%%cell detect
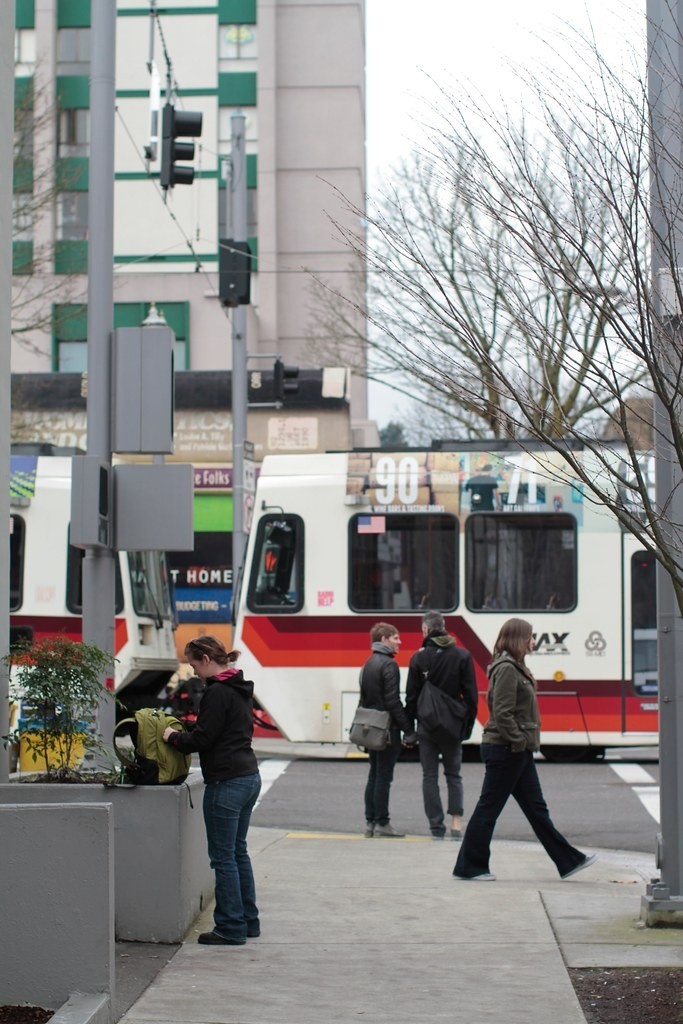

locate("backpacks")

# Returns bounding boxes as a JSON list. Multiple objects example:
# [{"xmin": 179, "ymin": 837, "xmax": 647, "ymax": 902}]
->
[{"xmin": 113, "ymin": 708, "xmax": 191, "ymax": 785}]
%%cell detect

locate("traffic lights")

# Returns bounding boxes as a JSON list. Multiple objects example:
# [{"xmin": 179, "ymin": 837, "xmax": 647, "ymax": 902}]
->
[{"xmin": 158, "ymin": 104, "xmax": 203, "ymax": 191}]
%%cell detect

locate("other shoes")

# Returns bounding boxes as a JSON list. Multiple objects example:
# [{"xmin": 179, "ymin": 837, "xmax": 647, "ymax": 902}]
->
[
  {"xmin": 198, "ymin": 932, "xmax": 247, "ymax": 945},
  {"xmin": 365, "ymin": 822, "xmax": 373, "ymax": 838},
  {"xmin": 561, "ymin": 854, "xmax": 598, "ymax": 879},
  {"xmin": 247, "ymin": 929, "xmax": 260, "ymax": 937},
  {"xmin": 450, "ymin": 829, "xmax": 462, "ymax": 841},
  {"xmin": 452, "ymin": 873, "xmax": 496, "ymax": 881},
  {"xmin": 374, "ymin": 822, "xmax": 406, "ymax": 838},
  {"xmin": 432, "ymin": 834, "xmax": 444, "ymax": 840}
]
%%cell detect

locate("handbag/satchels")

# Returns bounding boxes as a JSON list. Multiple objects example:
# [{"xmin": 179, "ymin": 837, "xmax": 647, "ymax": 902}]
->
[
  {"xmin": 349, "ymin": 706, "xmax": 392, "ymax": 752},
  {"xmin": 416, "ymin": 680, "xmax": 467, "ymax": 746}
]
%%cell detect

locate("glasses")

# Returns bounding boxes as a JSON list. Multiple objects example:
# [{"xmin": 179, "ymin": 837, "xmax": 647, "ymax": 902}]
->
[{"xmin": 189, "ymin": 641, "xmax": 211, "ymax": 660}]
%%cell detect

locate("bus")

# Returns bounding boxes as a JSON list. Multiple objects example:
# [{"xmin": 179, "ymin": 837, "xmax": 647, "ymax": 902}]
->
[
  {"xmin": 229, "ymin": 449, "xmax": 660, "ymax": 766},
  {"xmin": 10, "ymin": 455, "xmax": 180, "ymax": 737}
]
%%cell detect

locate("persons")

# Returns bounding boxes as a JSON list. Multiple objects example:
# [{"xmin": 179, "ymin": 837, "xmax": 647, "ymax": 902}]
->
[
  {"xmin": 465, "ymin": 464, "xmax": 500, "ymax": 513},
  {"xmin": 453, "ymin": 618, "xmax": 598, "ymax": 881},
  {"xmin": 163, "ymin": 636, "xmax": 261, "ymax": 944},
  {"xmin": 404, "ymin": 610, "xmax": 478, "ymax": 841},
  {"xmin": 358, "ymin": 623, "xmax": 410, "ymax": 838}
]
